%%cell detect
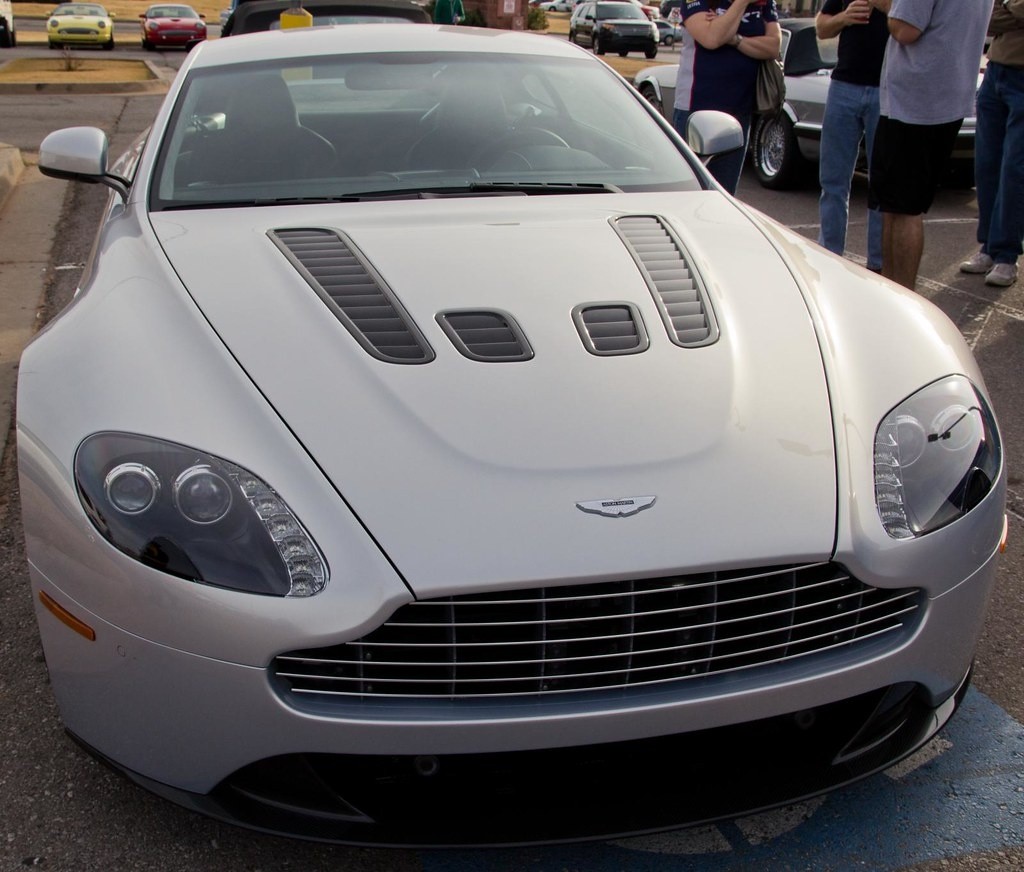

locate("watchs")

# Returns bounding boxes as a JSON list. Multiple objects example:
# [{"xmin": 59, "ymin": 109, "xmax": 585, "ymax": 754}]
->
[{"xmin": 733, "ymin": 34, "xmax": 741, "ymax": 48}]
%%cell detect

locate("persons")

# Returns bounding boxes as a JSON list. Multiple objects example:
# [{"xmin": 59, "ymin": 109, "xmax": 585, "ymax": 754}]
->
[
  {"xmin": 669, "ymin": 0, "xmax": 783, "ymax": 201},
  {"xmin": 814, "ymin": 0, "xmax": 1024, "ymax": 292}
]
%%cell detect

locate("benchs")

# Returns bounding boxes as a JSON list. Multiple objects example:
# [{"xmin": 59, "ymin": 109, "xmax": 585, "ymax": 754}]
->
[{"xmin": 295, "ymin": 110, "xmax": 430, "ymax": 177}]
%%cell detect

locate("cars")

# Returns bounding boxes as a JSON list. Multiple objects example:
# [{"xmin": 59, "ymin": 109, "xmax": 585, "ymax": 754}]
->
[
  {"xmin": 45, "ymin": 4, "xmax": 116, "ymax": 51},
  {"xmin": 220, "ymin": 0, "xmax": 434, "ymax": 39},
  {"xmin": 632, "ymin": 18, "xmax": 892, "ymax": 190},
  {"xmin": 139, "ymin": 4, "xmax": 207, "ymax": 52},
  {"xmin": 570, "ymin": 1, "xmax": 660, "ymax": 59},
  {"xmin": 15, "ymin": 26, "xmax": 1006, "ymax": 850},
  {"xmin": 654, "ymin": 21, "xmax": 685, "ymax": 46},
  {"xmin": 538, "ymin": 0, "xmax": 573, "ymax": 12}
]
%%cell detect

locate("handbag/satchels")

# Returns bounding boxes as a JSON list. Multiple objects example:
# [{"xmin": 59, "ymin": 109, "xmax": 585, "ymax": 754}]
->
[{"xmin": 756, "ymin": 58, "xmax": 787, "ymax": 115}]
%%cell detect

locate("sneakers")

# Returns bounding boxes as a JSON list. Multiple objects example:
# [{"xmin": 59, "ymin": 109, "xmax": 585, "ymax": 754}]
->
[
  {"xmin": 959, "ymin": 251, "xmax": 994, "ymax": 273},
  {"xmin": 985, "ymin": 262, "xmax": 1018, "ymax": 286}
]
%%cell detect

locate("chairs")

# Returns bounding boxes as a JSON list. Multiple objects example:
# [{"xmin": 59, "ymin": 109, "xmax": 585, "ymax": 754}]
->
[
  {"xmin": 186, "ymin": 74, "xmax": 338, "ymax": 184},
  {"xmin": 403, "ymin": 62, "xmax": 545, "ymax": 170}
]
